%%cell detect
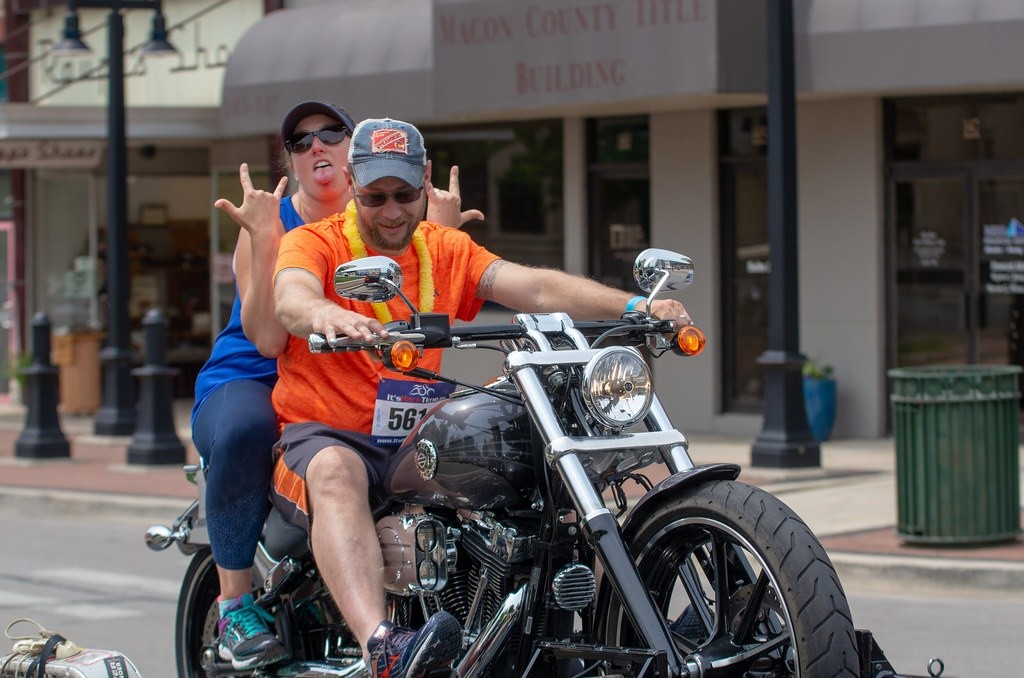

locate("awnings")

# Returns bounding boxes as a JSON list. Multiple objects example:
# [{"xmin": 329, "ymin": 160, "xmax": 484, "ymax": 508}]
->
[{"xmin": 220, "ymin": 0, "xmax": 1023, "ymax": 135}]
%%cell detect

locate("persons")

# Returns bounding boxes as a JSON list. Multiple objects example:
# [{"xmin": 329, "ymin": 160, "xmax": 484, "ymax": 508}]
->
[
  {"xmin": 190, "ymin": 100, "xmax": 485, "ymax": 669},
  {"xmin": 271, "ymin": 115, "xmax": 696, "ymax": 678}
]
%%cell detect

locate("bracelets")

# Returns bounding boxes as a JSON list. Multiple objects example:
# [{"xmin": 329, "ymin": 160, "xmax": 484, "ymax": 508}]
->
[{"xmin": 626, "ymin": 296, "xmax": 647, "ymax": 311}]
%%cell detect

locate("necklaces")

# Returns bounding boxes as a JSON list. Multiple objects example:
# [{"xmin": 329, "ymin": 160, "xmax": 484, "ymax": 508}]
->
[{"xmin": 341, "ymin": 197, "xmax": 434, "ymax": 326}]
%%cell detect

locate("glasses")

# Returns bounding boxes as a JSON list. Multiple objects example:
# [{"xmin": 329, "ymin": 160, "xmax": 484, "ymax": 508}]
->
[
  {"xmin": 286, "ymin": 123, "xmax": 353, "ymax": 154},
  {"xmin": 351, "ymin": 172, "xmax": 427, "ymax": 207}
]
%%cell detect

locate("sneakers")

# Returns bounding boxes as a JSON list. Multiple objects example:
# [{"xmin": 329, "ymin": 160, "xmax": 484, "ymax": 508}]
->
[
  {"xmin": 366, "ymin": 611, "xmax": 461, "ymax": 678},
  {"xmin": 214, "ymin": 592, "xmax": 291, "ymax": 669}
]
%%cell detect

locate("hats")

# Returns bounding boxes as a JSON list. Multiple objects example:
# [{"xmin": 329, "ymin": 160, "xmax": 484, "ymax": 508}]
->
[
  {"xmin": 347, "ymin": 117, "xmax": 427, "ymax": 190},
  {"xmin": 280, "ymin": 101, "xmax": 358, "ymax": 154}
]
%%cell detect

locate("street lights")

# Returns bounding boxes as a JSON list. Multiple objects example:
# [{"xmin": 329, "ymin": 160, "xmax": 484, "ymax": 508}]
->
[{"xmin": 52, "ymin": 0, "xmax": 178, "ymax": 435}]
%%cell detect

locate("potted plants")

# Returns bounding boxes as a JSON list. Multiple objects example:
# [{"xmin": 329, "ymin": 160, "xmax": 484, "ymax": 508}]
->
[{"xmin": 801, "ymin": 364, "xmax": 837, "ymax": 441}]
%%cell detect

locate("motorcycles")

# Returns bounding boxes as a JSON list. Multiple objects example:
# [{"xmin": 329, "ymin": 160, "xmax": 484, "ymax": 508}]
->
[{"xmin": 0, "ymin": 248, "xmax": 944, "ymax": 678}]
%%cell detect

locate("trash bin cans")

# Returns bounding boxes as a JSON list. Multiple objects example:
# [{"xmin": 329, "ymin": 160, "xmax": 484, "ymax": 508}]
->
[{"xmin": 887, "ymin": 365, "xmax": 1024, "ymax": 547}]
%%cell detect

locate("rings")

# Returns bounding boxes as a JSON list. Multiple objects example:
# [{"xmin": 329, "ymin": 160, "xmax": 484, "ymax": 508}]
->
[{"xmin": 680, "ymin": 315, "xmax": 686, "ymax": 318}]
